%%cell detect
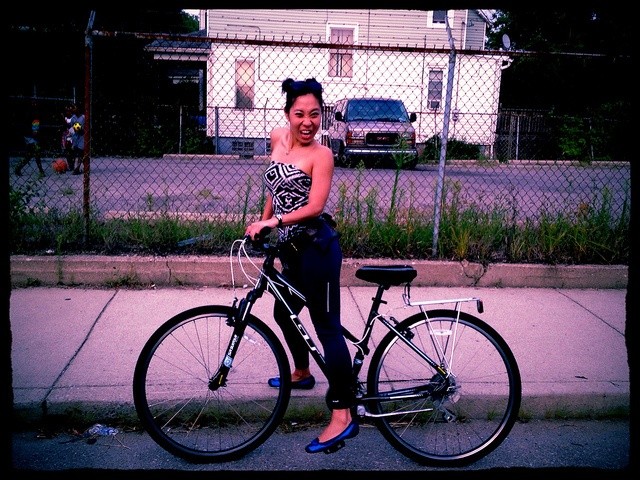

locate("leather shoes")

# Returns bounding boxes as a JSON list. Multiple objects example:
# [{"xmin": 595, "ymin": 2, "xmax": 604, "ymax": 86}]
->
[
  {"xmin": 305, "ymin": 417, "xmax": 359, "ymax": 453},
  {"xmin": 269, "ymin": 375, "xmax": 315, "ymax": 389}
]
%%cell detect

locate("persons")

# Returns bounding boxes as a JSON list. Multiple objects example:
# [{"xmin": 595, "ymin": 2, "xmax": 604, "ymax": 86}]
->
[
  {"xmin": 60, "ymin": 111, "xmax": 75, "ymax": 172},
  {"xmin": 70, "ymin": 102, "xmax": 93, "ymax": 175},
  {"xmin": 242, "ymin": 76, "xmax": 361, "ymax": 456},
  {"xmin": 14, "ymin": 100, "xmax": 47, "ymax": 177}
]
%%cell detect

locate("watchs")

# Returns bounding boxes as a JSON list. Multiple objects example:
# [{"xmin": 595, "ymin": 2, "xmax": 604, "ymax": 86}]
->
[{"xmin": 274, "ymin": 214, "xmax": 283, "ymax": 226}]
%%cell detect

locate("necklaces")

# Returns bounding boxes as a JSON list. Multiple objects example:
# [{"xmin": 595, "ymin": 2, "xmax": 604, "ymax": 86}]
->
[{"xmin": 286, "ymin": 140, "xmax": 299, "ymax": 156}]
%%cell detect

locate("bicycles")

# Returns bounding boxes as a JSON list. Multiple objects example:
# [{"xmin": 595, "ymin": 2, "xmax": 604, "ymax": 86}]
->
[{"xmin": 133, "ymin": 226, "xmax": 521, "ymax": 467}]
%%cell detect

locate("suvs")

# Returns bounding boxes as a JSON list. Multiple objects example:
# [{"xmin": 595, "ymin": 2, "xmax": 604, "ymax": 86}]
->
[{"xmin": 328, "ymin": 98, "xmax": 418, "ymax": 170}]
